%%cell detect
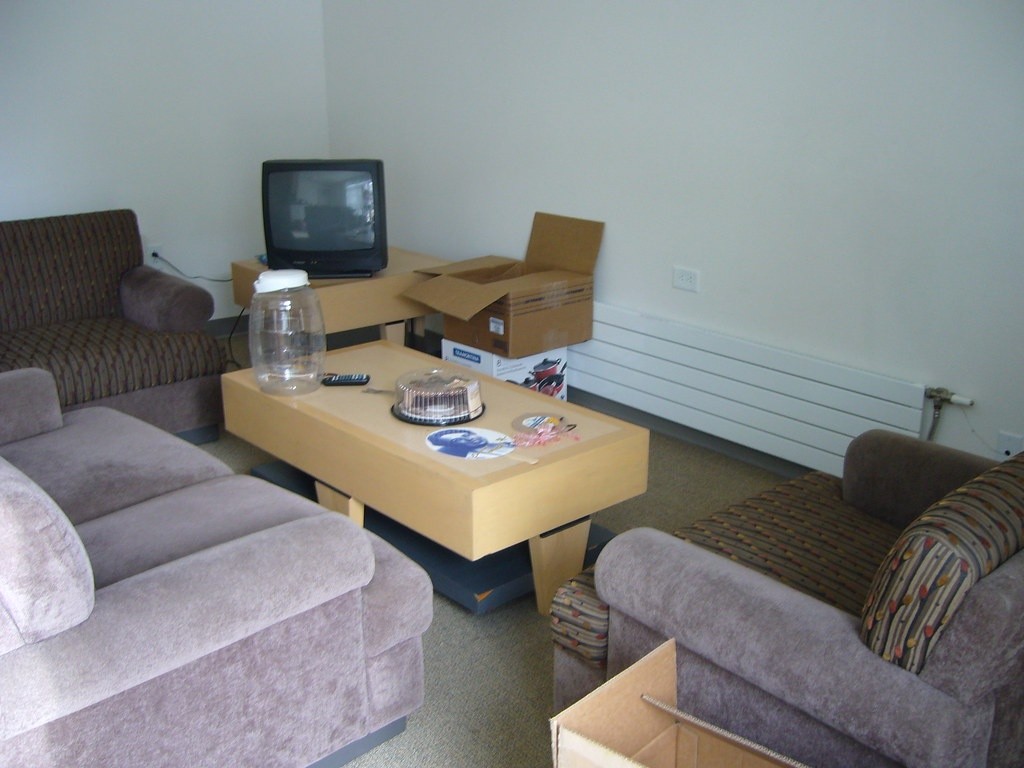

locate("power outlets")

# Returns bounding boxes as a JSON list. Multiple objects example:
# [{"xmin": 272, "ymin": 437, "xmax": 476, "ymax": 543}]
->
[
  {"xmin": 673, "ymin": 266, "xmax": 699, "ymax": 291},
  {"xmin": 146, "ymin": 246, "xmax": 163, "ymax": 272}
]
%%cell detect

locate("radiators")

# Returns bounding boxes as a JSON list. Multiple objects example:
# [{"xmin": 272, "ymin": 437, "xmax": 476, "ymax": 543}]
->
[{"xmin": 568, "ymin": 302, "xmax": 973, "ymax": 477}]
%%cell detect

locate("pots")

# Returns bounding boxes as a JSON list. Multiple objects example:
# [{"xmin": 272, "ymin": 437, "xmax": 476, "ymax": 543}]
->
[{"xmin": 505, "ymin": 358, "xmax": 567, "ymax": 397}]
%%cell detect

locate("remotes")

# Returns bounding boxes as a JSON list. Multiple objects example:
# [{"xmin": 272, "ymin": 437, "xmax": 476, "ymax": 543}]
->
[{"xmin": 322, "ymin": 374, "xmax": 371, "ymax": 386}]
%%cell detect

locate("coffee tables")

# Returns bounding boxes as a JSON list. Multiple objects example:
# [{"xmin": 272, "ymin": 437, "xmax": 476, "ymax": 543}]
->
[{"xmin": 221, "ymin": 340, "xmax": 649, "ymax": 614}]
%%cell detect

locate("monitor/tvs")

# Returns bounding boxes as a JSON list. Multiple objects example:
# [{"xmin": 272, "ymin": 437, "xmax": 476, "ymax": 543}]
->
[{"xmin": 262, "ymin": 157, "xmax": 390, "ymax": 278}]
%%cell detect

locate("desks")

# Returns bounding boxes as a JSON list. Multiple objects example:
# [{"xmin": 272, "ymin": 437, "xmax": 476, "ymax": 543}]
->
[{"xmin": 232, "ymin": 246, "xmax": 451, "ymax": 347}]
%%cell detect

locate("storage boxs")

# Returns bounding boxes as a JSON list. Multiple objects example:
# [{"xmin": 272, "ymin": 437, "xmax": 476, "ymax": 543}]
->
[
  {"xmin": 442, "ymin": 338, "xmax": 568, "ymax": 402},
  {"xmin": 401, "ymin": 212, "xmax": 604, "ymax": 359},
  {"xmin": 549, "ymin": 637, "xmax": 810, "ymax": 768}
]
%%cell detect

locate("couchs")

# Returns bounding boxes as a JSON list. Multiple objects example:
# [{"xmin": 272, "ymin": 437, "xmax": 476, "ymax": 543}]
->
[
  {"xmin": 548, "ymin": 428, "xmax": 1024, "ymax": 768},
  {"xmin": 0, "ymin": 368, "xmax": 433, "ymax": 767},
  {"xmin": 0, "ymin": 209, "xmax": 225, "ymax": 444}
]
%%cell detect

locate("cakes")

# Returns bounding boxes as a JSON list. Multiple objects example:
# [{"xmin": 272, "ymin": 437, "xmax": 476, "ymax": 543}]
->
[{"xmin": 399, "ymin": 375, "xmax": 483, "ymax": 422}]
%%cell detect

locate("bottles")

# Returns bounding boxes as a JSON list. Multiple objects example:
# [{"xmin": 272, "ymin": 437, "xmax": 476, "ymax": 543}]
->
[{"xmin": 247, "ymin": 269, "xmax": 328, "ymax": 399}]
